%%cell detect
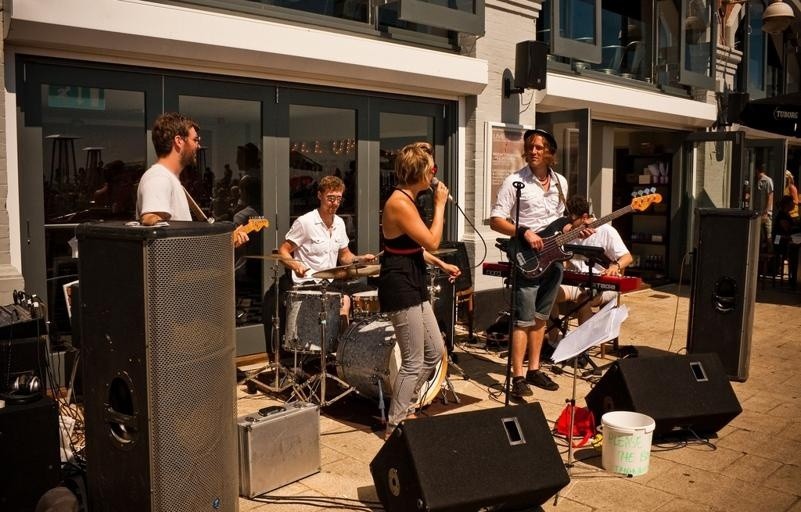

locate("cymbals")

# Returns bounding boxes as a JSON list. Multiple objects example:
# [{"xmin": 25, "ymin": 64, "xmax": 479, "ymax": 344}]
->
[
  {"xmin": 313, "ymin": 265, "xmax": 380, "ymax": 278},
  {"xmin": 427, "ymin": 249, "xmax": 457, "ymax": 256},
  {"xmin": 353, "ymin": 289, "xmax": 379, "ymax": 314}
]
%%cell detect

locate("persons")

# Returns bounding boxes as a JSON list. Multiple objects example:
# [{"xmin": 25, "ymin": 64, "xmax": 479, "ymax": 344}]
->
[
  {"xmin": 377, "ymin": 141, "xmax": 464, "ymax": 442},
  {"xmin": 784, "ymin": 168, "xmax": 800, "ymax": 223},
  {"xmin": 772, "ymin": 196, "xmax": 801, "ymax": 288},
  {"xmin": 183, "ymin": 163, "xmax": 241, "ymax": 221},
  {"xmin": 135, "ymin": 110, "xmax": 251, "ymax": 249},
  {"xmin": 491, "ymin": 126, "xmax": 598, "ymax": 400},
  {"xmin": 278, "ymin": 174, "xmax": 380, "ymax": 335},
  {"xmin": 289, "ymin": 160, "xmax": 357, "ymax": 216},
  {"xmin": 233, "ymin": 143, "xmax": 262, "ymax": 223},
  {"xmin": 93, "ymin": 161, "xmax": 138, "ymax": 220},
  {"xmin": 753, "ymin": 161, "xmax": 777, "ymax": 255},
  {"xmin": 546, "ymin": 193, "xmax": 636, "ymax": 369}
]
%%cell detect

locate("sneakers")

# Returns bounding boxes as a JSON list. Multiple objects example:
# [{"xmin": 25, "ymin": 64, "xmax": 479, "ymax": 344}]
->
[{"xmin": 512, "ymin": 370, "xmax": 559, "ymax": 395}]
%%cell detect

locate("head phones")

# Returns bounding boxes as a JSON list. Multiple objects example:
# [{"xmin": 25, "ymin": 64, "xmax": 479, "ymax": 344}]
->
[{"xmin": 0, "ymin": 375, "xmax": 42, "ymax": 404}]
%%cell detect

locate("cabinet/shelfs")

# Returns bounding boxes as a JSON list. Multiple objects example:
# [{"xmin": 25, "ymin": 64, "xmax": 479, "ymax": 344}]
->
[{"xmin": 621, "ymin": 149, "xmax": 680, "ymax": 283}]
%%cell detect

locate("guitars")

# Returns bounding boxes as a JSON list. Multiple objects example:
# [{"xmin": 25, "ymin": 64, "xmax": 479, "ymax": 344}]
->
[
  {"xmin": 234, "ymin": 216, "xmax": 269, "ymax": 245},
  {"xmin": 506, "ymin": 187, "xmax": 662, "ymax": 279}
]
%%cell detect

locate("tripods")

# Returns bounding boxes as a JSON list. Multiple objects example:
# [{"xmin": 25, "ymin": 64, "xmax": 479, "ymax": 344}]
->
[
  {"xmin": 240, "ymin": 260, "xmax": 469, "ymax": 424},
  {"xmin": 553, "ymin": 357, "xmax": 633, "ymax": 507}
]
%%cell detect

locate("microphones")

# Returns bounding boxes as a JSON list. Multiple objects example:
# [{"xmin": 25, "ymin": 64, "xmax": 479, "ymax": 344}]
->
[{"xmin": 432, "ymin": 177, "xmax": 457, "ymax": 206}]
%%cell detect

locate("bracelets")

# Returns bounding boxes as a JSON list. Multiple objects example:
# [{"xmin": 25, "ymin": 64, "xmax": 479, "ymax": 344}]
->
[
  {"xmin": 614, "ymin": 261, "xmax": 621, "ymax": 271},
  {"xmin": 519, "ymin": 225, "xmax": 529, "ymax": 236}
]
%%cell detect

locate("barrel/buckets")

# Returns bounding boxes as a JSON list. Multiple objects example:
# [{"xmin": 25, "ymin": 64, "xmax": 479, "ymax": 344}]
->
[{"xmin": 600, "ymin": 410, "xmax": 655, "ymax": 476}]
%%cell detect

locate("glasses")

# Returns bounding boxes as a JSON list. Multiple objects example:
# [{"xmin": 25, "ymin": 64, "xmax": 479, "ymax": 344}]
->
[
  {"xmin": 430, "ymin": 165, "xmax": 438, "ymax": 174},
  {"xmin": 327, "ymin": 195, "xmax": 344, "ymax": 202}
]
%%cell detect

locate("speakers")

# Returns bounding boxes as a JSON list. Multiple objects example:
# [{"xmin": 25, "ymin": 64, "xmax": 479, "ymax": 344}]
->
[
  {"xmin": 515, "ymin": 40, "xmax": 547, "ymax": 90},
  {"xmin": 584, "ymin": 350, "xmax": 743, "ymax": 445},
  {"xmin": 369, "ymin": 402, "xmax": 570, "ymax": 512},
  {"xmin": 0, "ymin": 396, "xmax": 62, "ymax": 512},
  {"xmin": 686, "ymin": 206, "xmax": 762, "ymax": 382},
  {"xmin": 727, "ymin": 91, "xmax": 749, "ymax": 120},
  {"xmin": 436, "ymin": 240, "xmax": 472, "ymax": 292},
  {"xmin": 0, "ymin": 334, "xmax": 47, "ymax": 400},
  {"xmin": 77, "ymin": 220, "xmax": 238, "ymax": 512}
]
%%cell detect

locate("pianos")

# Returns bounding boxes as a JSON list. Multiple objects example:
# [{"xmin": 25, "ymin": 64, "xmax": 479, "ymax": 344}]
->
[{"xmin": 484, "ymin": 261, "xmax": 640, "ymax": 292}]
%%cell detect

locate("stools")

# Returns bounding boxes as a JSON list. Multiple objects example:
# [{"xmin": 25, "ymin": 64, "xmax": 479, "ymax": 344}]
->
[
  {"xmin": 557, "ymin": 290, "xmax": 621, "ymax": 360},
  {"xmin": 455, "ymin": 286, "xmax": 478, "ymax": 339}
]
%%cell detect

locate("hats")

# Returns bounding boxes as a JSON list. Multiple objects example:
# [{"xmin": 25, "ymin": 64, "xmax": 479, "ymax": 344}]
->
[{"xmin": 524, "ymin": 127, "xmax": 557, "ymax": 148}]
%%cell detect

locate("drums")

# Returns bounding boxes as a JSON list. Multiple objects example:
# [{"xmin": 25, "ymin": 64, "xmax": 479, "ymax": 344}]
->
[
  {"xmin": 283, "ymin": 290, "xmax": 343, "ymax": 356},
  {"xmin": 336, "ymin": 314, "xmax": 449, "ymax": 407}
]
